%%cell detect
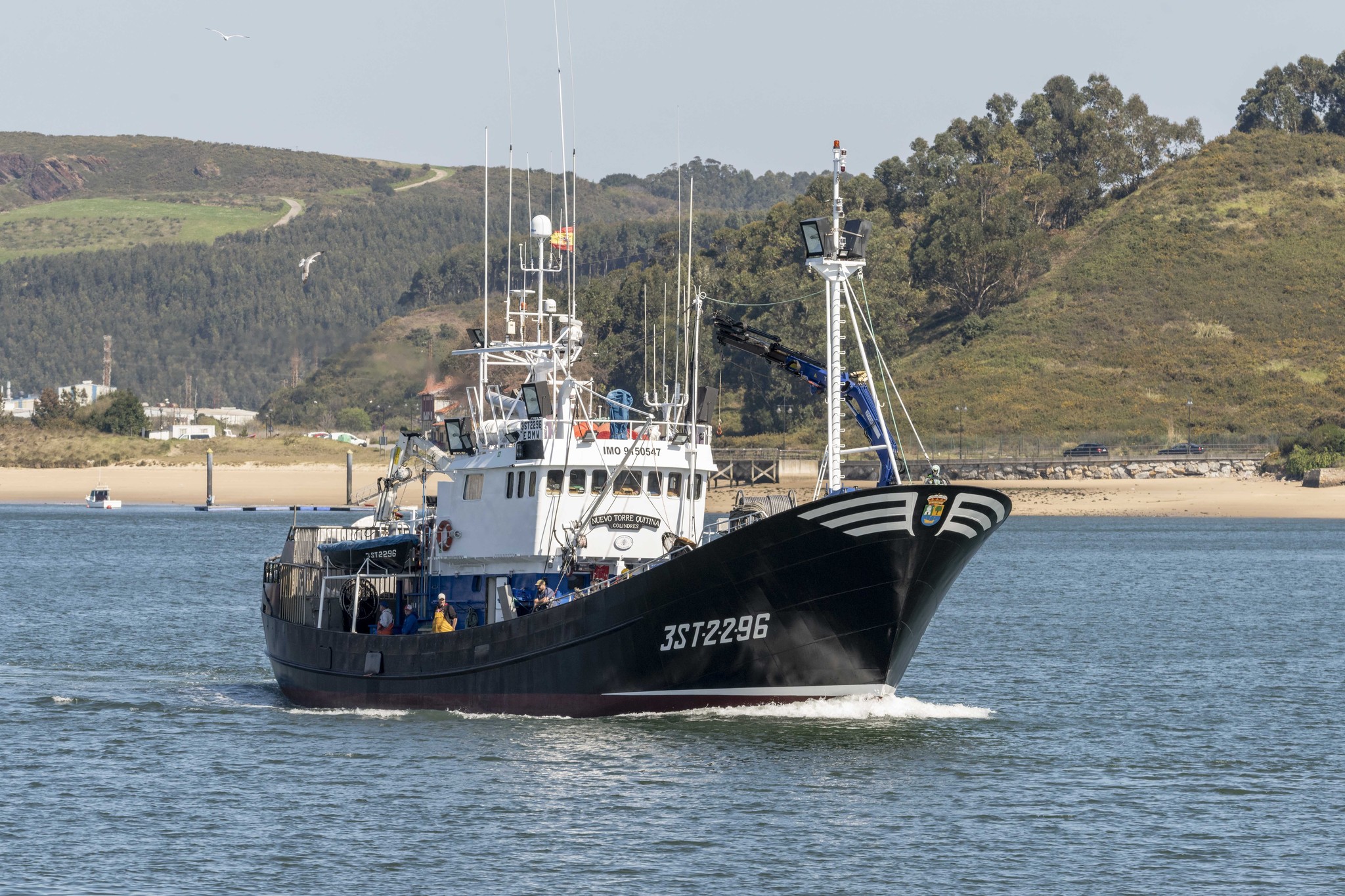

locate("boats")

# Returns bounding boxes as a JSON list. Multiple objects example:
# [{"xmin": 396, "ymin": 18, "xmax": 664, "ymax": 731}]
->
[
  {"xmin": 86, "ymin": 459, "xmax": 122, "ymax": 509},
  {"xmin": 259, "ymin": 4, "xmax": 1015, "ymax": 704}
]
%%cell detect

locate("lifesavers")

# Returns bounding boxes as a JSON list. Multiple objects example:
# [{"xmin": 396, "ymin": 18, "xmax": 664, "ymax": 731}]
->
[
  {"xmin": 437, "ymin": 520, "xmax": 452, "ymax": 551},
  {"xmin": 416, "ymin": 523, "xmax": 431, "ymax": 553}
]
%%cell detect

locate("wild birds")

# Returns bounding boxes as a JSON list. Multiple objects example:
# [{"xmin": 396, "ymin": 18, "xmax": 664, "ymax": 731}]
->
[
  {"xmin": 298, "ymin": 251, "xmax": 325, "ymax": 285},
  {"xmin": 204, "ymin": 28, "xmax": 250, "ymax": 41}
]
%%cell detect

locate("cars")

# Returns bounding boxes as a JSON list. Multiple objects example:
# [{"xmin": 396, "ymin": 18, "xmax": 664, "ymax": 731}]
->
[
  {"xmin": 1158, "ymin": 442, "xmax": 1205, "ymax": 455},
  {"xmin": 303, "ymin": 431, "xmax": 366, "ymax": 447}
]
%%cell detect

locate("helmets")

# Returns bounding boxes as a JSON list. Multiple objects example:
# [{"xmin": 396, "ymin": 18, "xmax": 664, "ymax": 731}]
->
[{"xmin": 621, "ymin": 569, "xmax": 632, "ymax": 577}]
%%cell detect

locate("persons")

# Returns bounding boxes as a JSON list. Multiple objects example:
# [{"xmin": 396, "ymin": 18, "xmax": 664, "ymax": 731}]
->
[
  {"xmin": 401, "ymin": 604, "xmax": 418, "ymax": 635},
  {"xmin": 923, "ymin": 465, "xmax": 946, "ymax": 484},
  {"xmin": 377, "ymin": 601, "xmax": 394, "ymax": 635},
  {"xmin": 534, "ymin": 579, "xmax": 557, "ymax": 612},
  {"xmin": 432, "ymin": 593, "xmax": 457, "ymax": 634}
]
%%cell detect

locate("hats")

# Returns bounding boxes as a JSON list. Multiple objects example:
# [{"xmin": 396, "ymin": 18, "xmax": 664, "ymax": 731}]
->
[
  {"xmin": 931, "ymin": 466, "xmax": 939, "ymax": 470},
  {"xmin": 379, "ymin": 601, "xmax": 388, "ymax": 608},
  {"xmin": 534, "ymin": 580, "xmax": 545, "ymax": 586},
  {"xmin": 405, "ymin": 604, "xmax": 412, "ymax": 610},
  {"xmin": 438, "ymin": 593, "xmax": 445, "ymax": 600}
]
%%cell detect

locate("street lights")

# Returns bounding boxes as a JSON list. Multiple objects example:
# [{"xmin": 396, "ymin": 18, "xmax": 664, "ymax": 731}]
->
[
  {"xmin": 775, "ymin": 396, "xmax": 794, "ymax": 446},
  {"xmin": 954, "ymin": 396, "xmax": 968, "ymax": 458},
  {"xmin": 1186, "ymin": 389, "xmax": 1193, "ymax": 455}
]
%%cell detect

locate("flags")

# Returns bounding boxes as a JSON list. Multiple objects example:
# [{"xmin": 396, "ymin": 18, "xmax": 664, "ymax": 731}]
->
[{"xmin": 548, "ymin": 226, "xmax": 575, "ymax": 252}]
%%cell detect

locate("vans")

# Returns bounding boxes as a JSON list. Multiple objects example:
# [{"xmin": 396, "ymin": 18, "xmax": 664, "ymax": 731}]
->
[{"xmin": 178, "ymin": 433, "xmax": 211, "ymax": 443}]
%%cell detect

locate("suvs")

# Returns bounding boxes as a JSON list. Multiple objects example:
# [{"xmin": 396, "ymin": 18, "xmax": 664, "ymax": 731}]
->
[{"xmin": 1063, "ymin": 443, "xmax": 1108, "ymax": 458}]
